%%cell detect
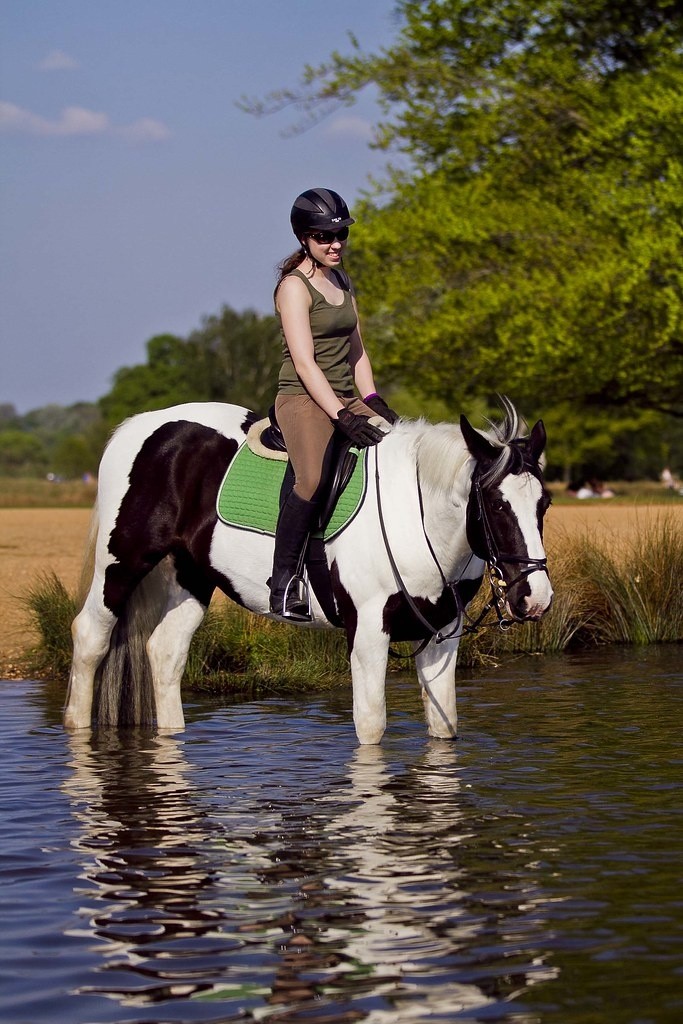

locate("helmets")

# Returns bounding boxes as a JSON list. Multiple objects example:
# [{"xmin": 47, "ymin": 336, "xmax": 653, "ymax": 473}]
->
[{"xmin": 290, "ymin": 188, "xmax": 355, "ymax": 238}]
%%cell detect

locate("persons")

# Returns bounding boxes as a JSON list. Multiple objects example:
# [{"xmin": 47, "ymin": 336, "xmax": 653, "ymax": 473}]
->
[{"xmin": 267, "ymin": 187, "xmax": 402, "ymax": 622}]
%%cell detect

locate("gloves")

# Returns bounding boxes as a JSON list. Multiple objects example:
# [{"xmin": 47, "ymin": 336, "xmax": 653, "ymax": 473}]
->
[
  {"xmin": 330, "ymin": 407, "xmax": 384, "ymax": 447},
  {"xmin": 363, "ymin": 393, "xmax": 403, "ymax": 425}
]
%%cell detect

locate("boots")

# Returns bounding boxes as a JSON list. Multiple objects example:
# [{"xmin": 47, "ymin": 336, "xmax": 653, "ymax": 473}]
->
[{"xmin": 266, "ymin": 490, "xmax": 310, "ymax": 615}]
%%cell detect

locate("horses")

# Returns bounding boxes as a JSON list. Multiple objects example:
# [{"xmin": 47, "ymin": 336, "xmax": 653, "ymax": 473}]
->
[{"xmin": 63, "ymin": 389, "xmax": 554, "ymax": 745}]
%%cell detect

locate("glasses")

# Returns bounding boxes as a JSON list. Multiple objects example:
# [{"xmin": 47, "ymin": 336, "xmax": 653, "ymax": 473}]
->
[{"xmin": 304, "ymin": 227, "xmax": 349, "ymax": 244}]
